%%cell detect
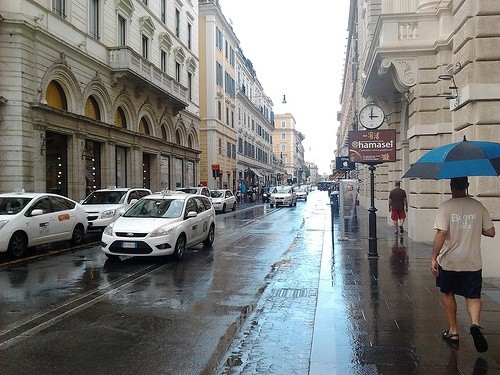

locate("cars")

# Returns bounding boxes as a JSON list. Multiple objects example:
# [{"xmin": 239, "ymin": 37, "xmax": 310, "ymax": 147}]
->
[
  {"xmin": 262, "ymin": 187, "xmax": 275, "ymax": 202},
  {"xmin": 100, "ymin": 189, "xmax": 216, "ymax": 262},
  {"xmin": 269, "ymin": 186, "xmax": 297, "ymax": 207},
  {"xmin": 0, "ymin": 188, "xmax": 89, "ymax": 259},
  {"xmin": 176, "ymin": 186, "xmax": 237, "ymax": 212},
  {"xmin": 80, "ymin": 185, "xmax": 152, "ymax": 233},
  {"xmin": 294, "ymin": 185, "xmax": 315, "ymax": 201}
]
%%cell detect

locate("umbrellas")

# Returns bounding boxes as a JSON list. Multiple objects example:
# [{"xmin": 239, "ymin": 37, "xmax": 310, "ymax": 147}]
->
[{"xmin": 401, "ymin": 134, "xmax": 500, "ymax": 198}]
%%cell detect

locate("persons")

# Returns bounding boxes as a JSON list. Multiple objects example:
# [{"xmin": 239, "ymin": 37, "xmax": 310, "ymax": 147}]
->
[
  {"xmin": 388, "ymin": 180, "xmax": 408, "ymax": 234},
  {"xmin": 430, "ymin": 177, "xmax": 495, "ymax": 353}
]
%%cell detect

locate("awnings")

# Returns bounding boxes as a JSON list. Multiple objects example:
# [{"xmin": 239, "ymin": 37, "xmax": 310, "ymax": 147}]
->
[{"xmin": 251, "ymin": 169, "xmax": 264, "ymax": 178}]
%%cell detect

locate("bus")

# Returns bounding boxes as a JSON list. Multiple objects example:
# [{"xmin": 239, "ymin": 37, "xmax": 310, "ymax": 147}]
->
[{"xmin": 317, "ymin": 181, "xmax": 336, "ymax": 191}]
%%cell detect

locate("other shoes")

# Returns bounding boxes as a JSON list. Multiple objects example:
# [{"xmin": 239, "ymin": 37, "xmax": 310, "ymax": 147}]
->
[
  {"xmin": 400, "ymin": 225, "xmax": 404, "ymax": 233},
  {"xmin": 394, "ymin": 230, "xmax": 398, "ymax": 234}
]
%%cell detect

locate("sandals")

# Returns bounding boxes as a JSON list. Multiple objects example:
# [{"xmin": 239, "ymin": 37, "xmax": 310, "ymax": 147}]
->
[
  {"xmin": 470, "ymin": 324, "xmax": 488, "ymax": 353},
  {"xmin": 442, "ymin": 329, "xmax": 459, "ymax": 343}
]
